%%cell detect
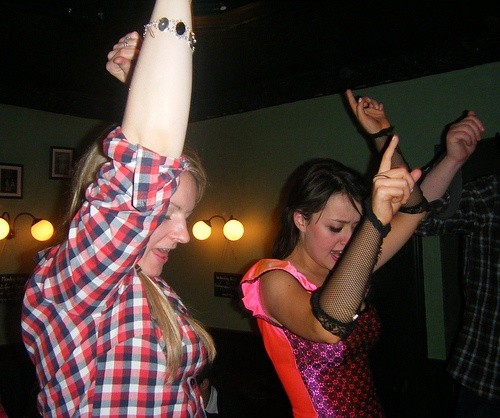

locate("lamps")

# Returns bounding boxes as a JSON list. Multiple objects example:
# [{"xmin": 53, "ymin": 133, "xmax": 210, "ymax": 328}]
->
[
  {"xmin": 0, "ymin": 211, "xmax": 54, "ymax": 242},
  {"xmin": 191, "ymin": 214, "xmax": 244, "ymax": 241}
]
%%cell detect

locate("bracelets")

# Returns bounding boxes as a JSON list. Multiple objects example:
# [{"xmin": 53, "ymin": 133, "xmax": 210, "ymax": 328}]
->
[{"xmin": 143, "ymin": 16, "xmax": 197, "ymax": 55}]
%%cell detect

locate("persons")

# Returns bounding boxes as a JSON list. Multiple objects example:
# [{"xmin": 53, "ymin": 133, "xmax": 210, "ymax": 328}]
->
[
  {"xmin": 21, "ymin": 0, "xmax": 216, "ymax": 418},
  {"xmin": 238, "ymin": 82, "xmax": 428, "ymax": 418},
  {"xmin": 413, "ymin": 109, "xmax": 500, "ymax": 418}
]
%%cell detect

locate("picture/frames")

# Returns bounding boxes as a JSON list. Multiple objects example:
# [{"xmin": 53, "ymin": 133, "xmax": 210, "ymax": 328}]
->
[
  {"xmin": 49, "ymin": 146, "xmax": 75, "ymax": 180},
  {"xmin": 0, "ymin": 163, "xmax": 24, "ymax": 199}
]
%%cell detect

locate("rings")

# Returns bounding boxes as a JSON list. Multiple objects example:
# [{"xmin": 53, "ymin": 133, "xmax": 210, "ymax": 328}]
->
[
  {"xmin": 124, "ymin": 33, "xmax": 132, "ymax": 39},
  {"xmin": 124, "ymin": 40, "xmax": 128, "ymax": 47}
]
